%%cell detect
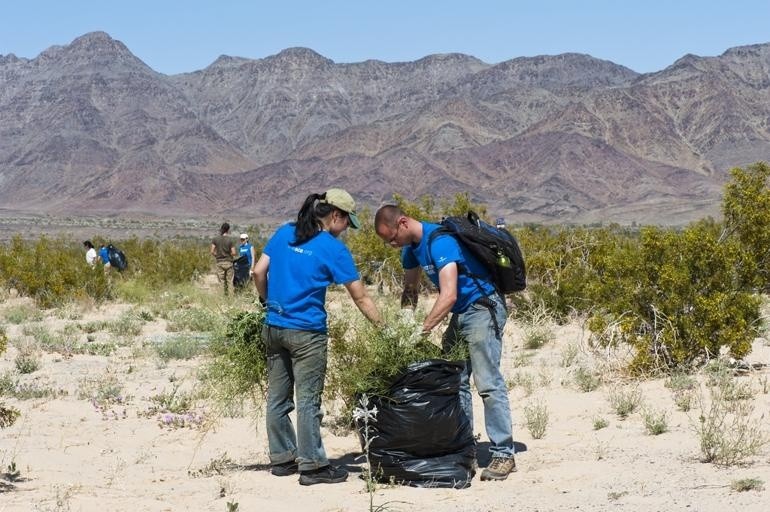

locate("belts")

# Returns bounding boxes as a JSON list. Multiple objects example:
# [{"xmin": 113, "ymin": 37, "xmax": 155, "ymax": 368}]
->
[{"xmin": 475, "ymin": 297, "xmax": 498, "ymax": 308}]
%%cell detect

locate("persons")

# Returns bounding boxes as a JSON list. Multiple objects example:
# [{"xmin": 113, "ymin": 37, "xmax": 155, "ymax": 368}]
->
[
  {"xmin": 252, "ymin": 187, "xmax": 396, "ymax": 490},
  {"xmin": 82, "ymin": 240, "xmax": 98, "ymax": 267},
  {"xmin": 239, "ymin": 233, "xmax": 256, "ymax": 284},
  {"xmin": 370, "ymin": 203, "xmax": 519, "ymax": 484},
  {"xmin": 91, "ymin": 243, "xmax": 114, "ymax": 277},
  {"xmin": 210, "ymin": 221, "xmax": 237, "ymax": 297}
]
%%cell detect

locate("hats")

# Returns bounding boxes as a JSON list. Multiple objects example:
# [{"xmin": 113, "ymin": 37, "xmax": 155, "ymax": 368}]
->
[
  {"xmin": 318, "ymin": 188, "xmax": 360, "ymax": 229},
  {"xmin": 240, "ymin": 234, "xmax": 249, "ymax": 239}
]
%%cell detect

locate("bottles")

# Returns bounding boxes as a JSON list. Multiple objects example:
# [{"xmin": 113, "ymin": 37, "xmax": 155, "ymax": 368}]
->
[{"xmin": 495, "ymin": 247, "xmax": 510, "ymax": 267}]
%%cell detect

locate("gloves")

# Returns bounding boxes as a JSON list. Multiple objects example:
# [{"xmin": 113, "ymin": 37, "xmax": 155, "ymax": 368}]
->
[{"xmin": 377, "ymin": 308, "xmax": 431, "ymax": 345}]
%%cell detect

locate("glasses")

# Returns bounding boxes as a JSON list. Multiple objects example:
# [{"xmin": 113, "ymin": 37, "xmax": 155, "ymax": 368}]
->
[{"xmin": 383, "ymin": 223, "xmax": 401, "ymax": 249}]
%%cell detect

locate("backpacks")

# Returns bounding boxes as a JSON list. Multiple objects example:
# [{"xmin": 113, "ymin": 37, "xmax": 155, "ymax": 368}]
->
[
  {"xmin": 107, "ymin": 244, "xmax": 127, "ymax": 269},
  {"xmin": 425, "ymin": 210, "xmax": 527, "ymax": 293}
]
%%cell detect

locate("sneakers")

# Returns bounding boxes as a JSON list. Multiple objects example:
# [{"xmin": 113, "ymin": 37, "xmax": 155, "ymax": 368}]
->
[
  {"xmin": 479, "ymin": 455, "xmax": 518, "ymax": 481},
  {"xmin": 298, "ymin": 466, "xmax": 348, "ymax": 485},
  {"xmin": 270, "ymin": 462, "xmax": 298, "ymax": 476}
]
